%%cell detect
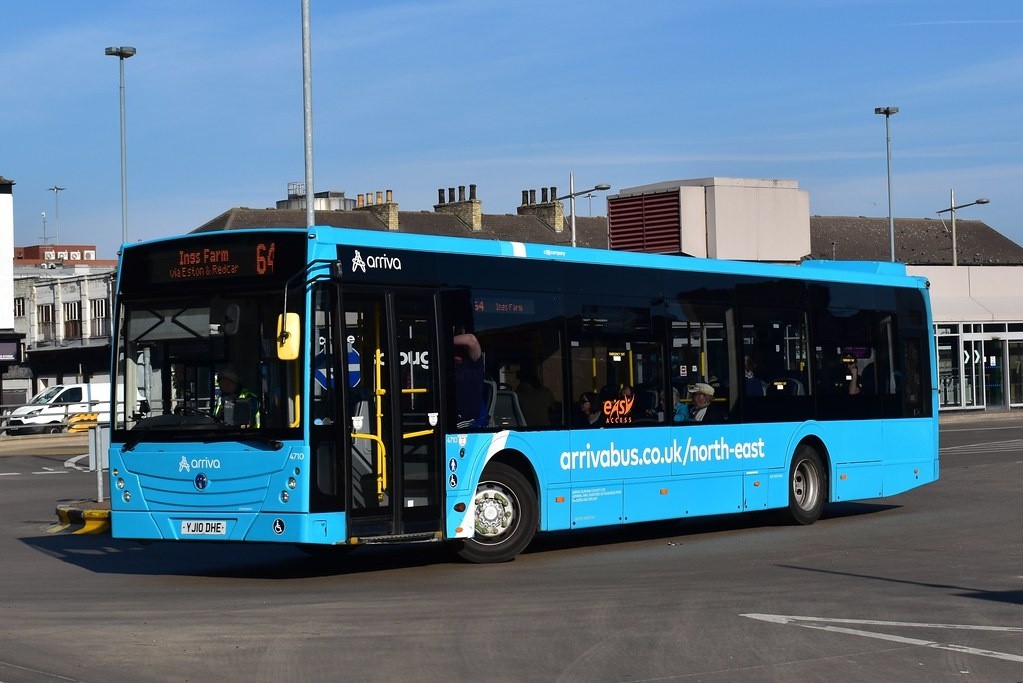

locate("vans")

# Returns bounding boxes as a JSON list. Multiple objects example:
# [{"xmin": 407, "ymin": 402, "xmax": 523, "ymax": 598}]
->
[{"xmin": 5, "ymin": 382, "xmax": 152, "ymax": 436}]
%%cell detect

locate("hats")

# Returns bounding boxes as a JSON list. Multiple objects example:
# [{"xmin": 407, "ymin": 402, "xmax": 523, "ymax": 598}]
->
[{"xmin": 688, "ymin": 383, "xmax": 714, "ymax": 396}]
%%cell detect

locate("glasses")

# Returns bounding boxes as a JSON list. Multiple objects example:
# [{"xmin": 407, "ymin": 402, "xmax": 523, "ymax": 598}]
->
[{"xmin": 217, "ymin": 377, "xmax": 227, "ymax": 383}]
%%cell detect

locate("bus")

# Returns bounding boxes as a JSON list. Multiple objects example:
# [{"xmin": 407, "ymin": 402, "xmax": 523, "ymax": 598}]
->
[{"xmin": 107, "ymin": 223, "xmax": 944, "ymax": 565}]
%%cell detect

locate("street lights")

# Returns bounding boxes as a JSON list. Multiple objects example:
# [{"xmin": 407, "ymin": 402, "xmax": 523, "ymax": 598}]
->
[
  {"xmin": 874, "ymin": 105, "xmax": 900, "ymax": 260},
  {"xmin": 555, "ymin": 171, "xmax": 611, "ymax": 248},
  {"xmin": 104, "ymin": 46, "xmax": 137, "ymax": 243},
  {"xmin": 936, "ymin": 188, "xmax": 990, "ymax": 266}
]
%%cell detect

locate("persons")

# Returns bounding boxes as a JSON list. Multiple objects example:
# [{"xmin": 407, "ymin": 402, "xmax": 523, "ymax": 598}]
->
[
  {"xmin": 348, "ymin": 333, "xmax": 486, "ymax": 426},
  {"xmin": 745, "ymin": 355, "xmax": 768, "ymax": 395},
  {"xmin": 830, "ymin": 354, "xmax": 868, "ymax": 406},
  {"xmin": 619, "ymin": 386, "xmax": 645, "ymax": 414},
  {"xmin": 689, "ymin": 374, "xmax": 720, "ymax": 421},
  {"xmin": 571, "ymin": 392, "xmax": 606, "ymax": 429},
  {"xmin": 212, "ymin": 363, "xmax": 260, "ymax": 430},
  {"xmin": 519, "ymin": 364, "xmax": 561, "ymax": 419},
  {"xmin": 656, "ymin": 387, "xmax": 687, "ymax": 423}
]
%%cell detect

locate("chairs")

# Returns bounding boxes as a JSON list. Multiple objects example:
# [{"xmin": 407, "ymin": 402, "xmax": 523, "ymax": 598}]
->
[
  {"xmin": 485, "ymin": 379, "xmax": 528, "ymax": 425},
  {"xmin": 644, "ymin": 362, "xmax": 875, "ymax": 423}
]
